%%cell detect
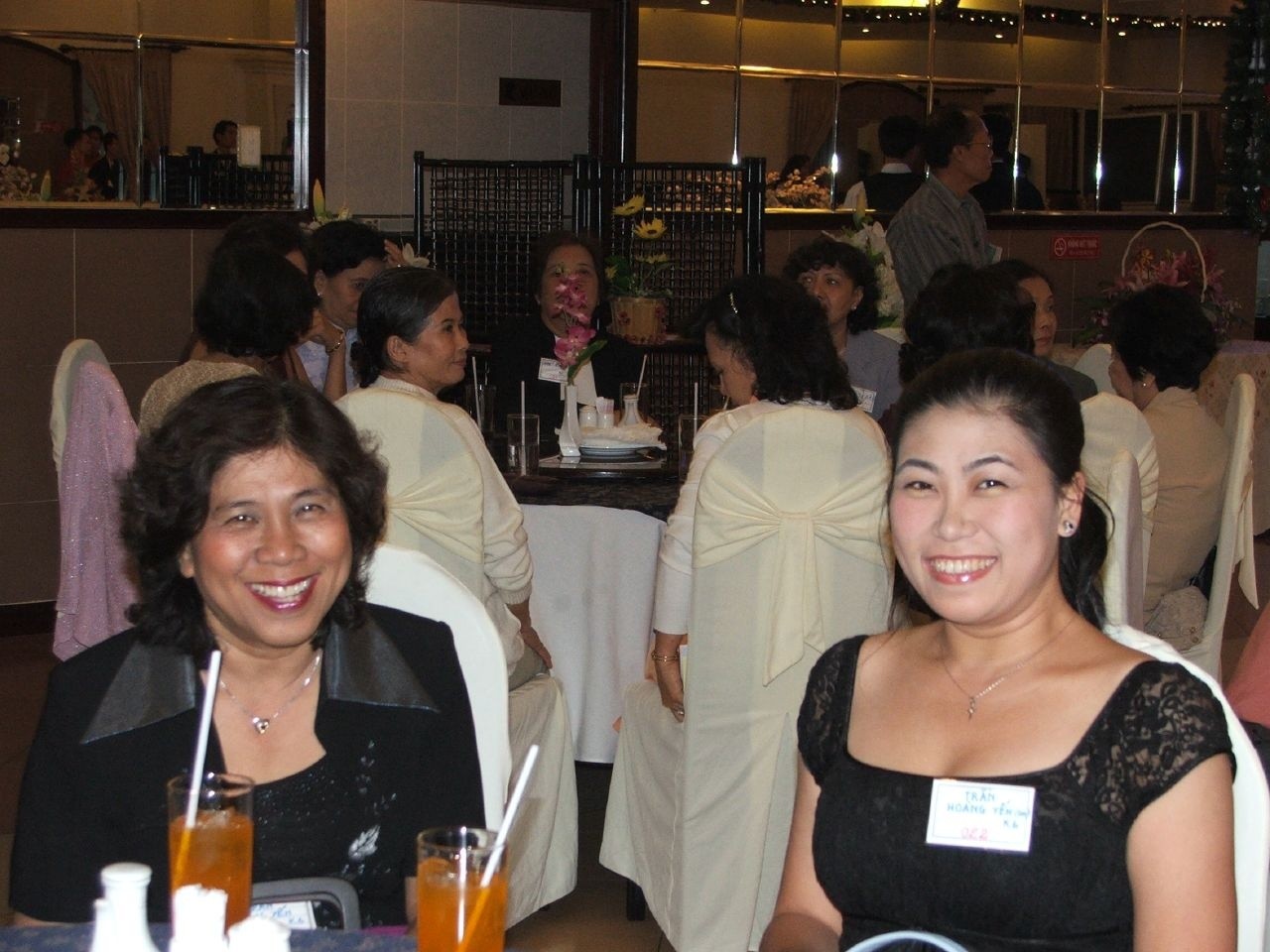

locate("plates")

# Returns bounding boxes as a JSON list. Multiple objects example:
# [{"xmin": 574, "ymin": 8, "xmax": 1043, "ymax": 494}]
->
[{"xmin": 557, "ymin": 441, "xmax": 667, "ymax": 458}]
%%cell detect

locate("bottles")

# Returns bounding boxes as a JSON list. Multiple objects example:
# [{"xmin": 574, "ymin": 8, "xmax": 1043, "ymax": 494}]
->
[
  {"xmin": 90, "ymin": 862, "xmax": 161, "ymax": 952},
  {"xmin": 619, "ymin": 395, "xmax": 645, "ymax": 425}
]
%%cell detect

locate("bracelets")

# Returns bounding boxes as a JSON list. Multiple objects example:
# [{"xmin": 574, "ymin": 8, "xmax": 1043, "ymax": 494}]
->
[
  {"xmin": 651, "ymin": 649, "xmax": 681, "ymax": 662},
  {"xmin": 325, "ymin": 330, "xmax": 346, "ymax": 355}
]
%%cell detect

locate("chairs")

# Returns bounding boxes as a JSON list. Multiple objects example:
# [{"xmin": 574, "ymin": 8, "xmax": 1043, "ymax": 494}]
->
[
  {"xmin": 1060, "ymin": 333, "xmax": 1270, "ymax": 952},
  {"xmin": 594, "ymin": 404, "xmax": 897, "ymax": 952},
  {"xmin": 361, "ymin": 542, "xmax": 581, "ymax": 935},
  {"xmin": 48, "ymin": 333, "xmax": 117, "ymax": 502}
]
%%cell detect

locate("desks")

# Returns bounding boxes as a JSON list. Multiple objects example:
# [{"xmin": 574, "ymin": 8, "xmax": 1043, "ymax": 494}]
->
[
  {"xmin": 492, "ymin": 444, "xmax": 688, "ymax": 766},
  {"xmin": 1041, "ymin": 339, "xmax": 1270, "ymax": 544}
]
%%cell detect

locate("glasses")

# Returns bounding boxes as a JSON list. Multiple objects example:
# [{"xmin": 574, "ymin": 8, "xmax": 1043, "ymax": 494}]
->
[{"xmin": 960, "ymin": 136, "xmax": 994, "ymax": 151}]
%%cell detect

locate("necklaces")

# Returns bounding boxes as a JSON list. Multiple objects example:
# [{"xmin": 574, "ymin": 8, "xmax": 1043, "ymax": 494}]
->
[
  {"xmin": 216, "ymin": 647, "xmax": 325, "ymax": 733},
  {"xmin": 935, "ymin": 614, "xmax": 1084, "ymax": 721}
]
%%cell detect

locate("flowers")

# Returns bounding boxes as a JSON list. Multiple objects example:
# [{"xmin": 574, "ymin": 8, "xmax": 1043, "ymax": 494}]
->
[
  {"xmin": 819, "ymin": 182, "xmax": 908, "ymax": 331},
  {"xmin": 761, "ymin": 163, "xmax": 837, "ymax": 212},
  {"xmin": 600, "ymin": 193, "xmax": 677, "ymax": 301},
  {"xmin": 293, "ymin": 177, "xmax": 355, "ymax": 239},
  {"xmin": 544, "ymin": 256, "xmax": 612, "ymax": 390},
  {"xmin": 1077, "ymin": 249, "xmax": 1256, "ymax": 344},
  {"xmin": 0, "ymin": 141, "xmax": 106, "ymax": 204}
]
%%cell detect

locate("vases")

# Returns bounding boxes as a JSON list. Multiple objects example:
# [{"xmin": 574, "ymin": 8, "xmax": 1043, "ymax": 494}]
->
[
  {"xmin": 607, "ymin": 294, "xmax": 670, "ymax": 349},
  {"xmin": 556, "ymin": 385, "xmax": 583, "ymax": 457}
]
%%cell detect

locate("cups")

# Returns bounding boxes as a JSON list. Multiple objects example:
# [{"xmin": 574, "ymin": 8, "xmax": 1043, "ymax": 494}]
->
[
  {"xmin": 709, "ymin": 408, "xmax": 732, "ymax": 417},
  {"xmin": 506, "ymin": 414, "xmax": 540, "ymax": 477},
  {"xmin": 620, "ymin": 383, "xmax": 648, "ymax": 423},
  {"xmin": 678, "ymin": 415, "xmax": 708, "ymax": 489},
  {"xmin": 464, "ymin": 384, "xmax": 496, "ymax": 443},
  {"xmin": 168, "ymin": 773, "xmax": 254, "ymax": 934},
  {"xmin": 417, "ymin": 828, "xmax": 506, "ymax": 952}
]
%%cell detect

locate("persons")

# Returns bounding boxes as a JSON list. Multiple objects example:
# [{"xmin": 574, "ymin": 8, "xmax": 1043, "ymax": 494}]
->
[
  {"xmin": 781, "ymin": 107, "xmax": 1230, "ymax": 620},
  {"xmin": 7, "ymin": 372, "xmax": 488, "ymax": 927},
  {"xmin": 128, "ymin": 217, "xmax": 628, "ymax": 454},
  {"xmin": 349, "ymin": 266, "xmax": 554, "ymax": 691},
  {"xmin": 67, "ymin": 120, "xmax": 295, "ymax": 204},
  {"xmin": 758, "ymin": 343, "xmax": 1238, "ymax": 952},
  {"xmin": 652, "ymin": 273, "xmax": 858, "ymax": 724}
]
